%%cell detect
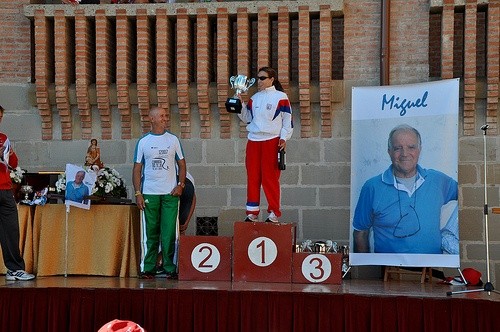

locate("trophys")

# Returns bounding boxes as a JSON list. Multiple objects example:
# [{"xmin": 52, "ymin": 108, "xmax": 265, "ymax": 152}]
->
[{"xmin": 225, "ymin": 75, "xmax": 255, "ymax": 114}]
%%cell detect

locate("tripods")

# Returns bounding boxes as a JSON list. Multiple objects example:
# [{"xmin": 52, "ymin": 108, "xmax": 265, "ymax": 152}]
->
[{"xmin": 446, "ymin": 134, "xmax": 500, "ymax": 295}]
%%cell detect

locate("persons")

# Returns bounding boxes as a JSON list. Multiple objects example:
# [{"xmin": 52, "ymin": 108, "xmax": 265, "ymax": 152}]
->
[
  {"xmin": 353, "ymin": 125, "xmax": 458, "ymax": 253},
  {"xmin": 237, "ymin": 66, "xmax": 294, "ymax": 222},
  {"xmin": 87, "ymin": 139, "xmax": 100, "ymax": 166},
  {"xmin": 0, "ymin": 106, "xmax": 35, "ymax": 281},
  {"xmin": 66, "ymin": 171, "xmax": 89, "ymax": 205},
  {"xmin": 132, "ymin": 108, "xmax": 186, "ymax": 278}
]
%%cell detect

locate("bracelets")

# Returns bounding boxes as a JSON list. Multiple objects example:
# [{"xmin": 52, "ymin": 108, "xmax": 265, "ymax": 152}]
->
[
  {"xmin": 135, "ymin": 191, "xmax": 140, "ymax": 196},
  {"xmin": 178, "ymin": 182, "xmax": 185, "ymax": 188}
]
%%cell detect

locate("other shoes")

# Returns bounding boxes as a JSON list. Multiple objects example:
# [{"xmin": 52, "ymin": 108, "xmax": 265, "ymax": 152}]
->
[
  {"xmin": 142, "ymin": 272, "xmax": 155, "ymax": 278},
  {"xmin": 167, "ymin": 271, "xmax": 177, "ymax": 279}
]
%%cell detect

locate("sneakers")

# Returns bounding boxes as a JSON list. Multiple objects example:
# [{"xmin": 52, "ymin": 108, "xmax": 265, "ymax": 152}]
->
[
  {"xmin": 244, "ymin": 214, "xmax": 260, "ymax": 222},
  {"xmin": 5, "ymin": 268, "xmax": 35, "ymax": 281},
  {"xmin": 265, "ymin": 212, "xmax": 278, "ymax": 222}
]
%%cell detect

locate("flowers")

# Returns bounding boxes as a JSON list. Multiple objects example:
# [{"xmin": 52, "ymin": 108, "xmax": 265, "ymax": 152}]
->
[
  {"xmin": 14, "ymin": 167, "xmax": 28, "ymax": 183},
  {"xmin": 55, "ymin": 164, "xmax": 128, "ymax": 200}
]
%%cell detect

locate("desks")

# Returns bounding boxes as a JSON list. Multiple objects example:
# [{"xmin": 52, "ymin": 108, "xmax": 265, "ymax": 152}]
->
[
  {"xmin": 0, "ymin": 205, "xmax": 34, "ymax": 274},
  {"xmin": 32, "ymin": 204, "xmax": 141, "ymax": 278}
]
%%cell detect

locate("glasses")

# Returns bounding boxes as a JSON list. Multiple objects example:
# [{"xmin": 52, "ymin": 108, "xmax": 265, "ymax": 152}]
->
[
  {"xmin": 257, "ymin": 76, "xmax": 271, "ymax": 80},
  {"xmin": 393, "ymin": 205, "xmax": 421, "ymax": 238}
]
